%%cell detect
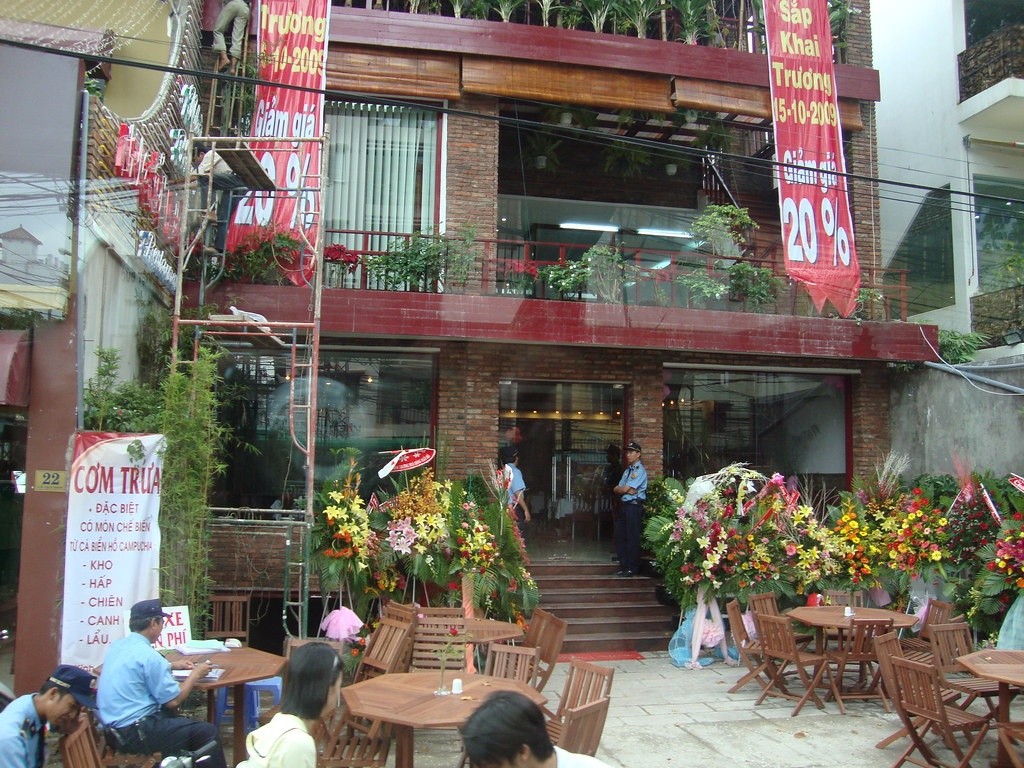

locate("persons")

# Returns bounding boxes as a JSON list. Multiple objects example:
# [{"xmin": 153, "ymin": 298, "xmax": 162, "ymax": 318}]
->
[
  {"xmin": 458, "ymin": 690, "xmax": 611, "ymax": 768},
  {"xmin": 234, "ymin": 643, "xmax": 345, "ymax": 768},
  {"xmin": 613, "ymin": 443, "xmax": 648, "ymax": 577},
  {"xmin": 211, "ymin": 0, "xmax": 250, "ymax": 75},
  {"xmin": 500, "ymin": 447, "xmax": 531, "ymax": 545},
  {"xmin": 0, "ymin": 663, "xmax": 100, "ymax": 768},
  {"xmin": 602, "ymin": 445, "xmax": 623, "ymax": 561},
  {"xmin": 94, "ymin": 598, "xmax": 228, "ymax": 768},
  {"xmin": 166, "ymin": 140, "xmax": 249, "ymax": 257}
]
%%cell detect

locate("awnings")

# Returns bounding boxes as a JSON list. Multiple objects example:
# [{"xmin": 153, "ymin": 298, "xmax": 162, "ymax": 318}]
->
[{"xmin": 0, "ymin": 328, "xmax": 32, "ymax": 407}]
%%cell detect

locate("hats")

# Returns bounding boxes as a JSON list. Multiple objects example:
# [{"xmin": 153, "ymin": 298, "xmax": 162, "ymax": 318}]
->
[
  {"xmin": 46, "ymin": 665, "xmax": 100, "ymax": 709},
  {"xmin": 501, "ymin": 446, "xmax": 519, "ymax": 459},
  {"xmin": 603, "ymin": 445, "xmax": 620, "ymax": 453},
  {"xmin": 624, "ymin": 442, "xmax": 642, "ymax": 453},
  {"xmin": 131, "ymin": 599, "xmax": 171, "ymax": 619}
]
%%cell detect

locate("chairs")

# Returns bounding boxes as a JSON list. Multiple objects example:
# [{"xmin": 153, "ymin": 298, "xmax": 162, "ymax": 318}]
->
[
  {"xmin": 204, "ymin": 594, "xmax": 615, "ymax": 768},
  {"xmin": 726, "ymin": 590, "xmax": 1020, "ymax": 768},
  {"xmin": 60, "ymin": 718, "xmax": 162, "ymax": 768}
]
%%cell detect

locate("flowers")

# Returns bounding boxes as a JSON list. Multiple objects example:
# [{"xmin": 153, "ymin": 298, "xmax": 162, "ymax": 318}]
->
[
  {"xmin": 500, "ymin": 261, "xmax": 538, "ymax": 297},
  {"xmin": 647, "ymin": 271, "xmax": 669, "ymax": 307},
  {"xmin": 321, "ymin": 456, "xmax": 537, "ymax": 656},
  {"xmin": 324, "ymin": 244, "xmax": 359, "ymax": 274},
  {"xmin": 642, "ymin": 469, "xmax": 1024, "ymax": 650},
  {"xmin": 438, "ymin": 628, "xmax": 460, "ymax": 662}
]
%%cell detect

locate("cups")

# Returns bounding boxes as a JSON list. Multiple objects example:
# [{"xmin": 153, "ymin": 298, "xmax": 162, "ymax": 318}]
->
[
  {"xmin": 452, "ymin": 679, "xmax": 463, "ymax": 694},
  {"xmin": 845, "ymin": 607, "xmax": 852, "ymax": 616}
]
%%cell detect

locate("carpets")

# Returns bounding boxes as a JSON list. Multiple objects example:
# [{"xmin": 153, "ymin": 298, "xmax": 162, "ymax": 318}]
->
[{"xmin": 555, "ymin": 650, "xmax": 646, "ymax": 662}]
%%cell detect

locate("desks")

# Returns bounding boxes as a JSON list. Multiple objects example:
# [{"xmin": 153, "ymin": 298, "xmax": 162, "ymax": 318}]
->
[
  {"xmin": 955, "ymin": 647, "xmax": 1024, "ymax": 768},
  {"xmin": 342, "ymin": 670, "xmax": 548, "ymax": 768},
  {"xmin": 93, "ymin": 646, "xmax": 287, "ymax": 768},
  {"xmin": 786, "ymin": 606, "xmax": 919, "ymax": 702},
  {"xmin": 466, "ymin": 618, "xmax": 524, "ymax": 644}
]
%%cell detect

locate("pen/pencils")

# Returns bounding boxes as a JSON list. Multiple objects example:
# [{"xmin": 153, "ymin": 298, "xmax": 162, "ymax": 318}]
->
[{"xmin": 207, "ymin": 664, "xmax": 221, "ymax": 667}]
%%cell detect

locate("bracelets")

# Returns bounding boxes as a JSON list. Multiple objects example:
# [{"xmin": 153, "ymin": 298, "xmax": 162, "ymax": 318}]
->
[{"xmin": 174, "ymin": 180, "xmax": 177, "ymax": 184}]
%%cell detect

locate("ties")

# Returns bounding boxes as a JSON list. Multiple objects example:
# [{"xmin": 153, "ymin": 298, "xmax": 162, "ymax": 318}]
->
[
  {"xmin": 625, "ymin": 468, "xmax": 631, "ymax": 488},
  {"xmin": 36, "ymin": 725, "xmax": 46, "ymax": 768}
]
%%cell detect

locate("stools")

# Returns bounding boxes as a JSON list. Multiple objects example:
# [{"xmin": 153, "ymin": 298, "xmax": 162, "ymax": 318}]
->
[
  {"xmin": 244, "ymin": 677, "xmax": 283, "ymax": 736},
  {"xmin": 216, "ymin": 683, "xmax": 261, "ymax": 739}
]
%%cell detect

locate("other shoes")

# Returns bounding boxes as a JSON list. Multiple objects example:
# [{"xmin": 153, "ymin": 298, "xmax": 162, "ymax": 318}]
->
[{"xmin": 619, "ymin": 570, "xmax": 637, "ymax": 577}]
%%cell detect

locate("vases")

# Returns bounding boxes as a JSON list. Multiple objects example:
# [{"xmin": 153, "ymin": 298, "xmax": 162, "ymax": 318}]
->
[{"xmin": 435, "ymin": 664, "xmax": 450, "ymax": 696}]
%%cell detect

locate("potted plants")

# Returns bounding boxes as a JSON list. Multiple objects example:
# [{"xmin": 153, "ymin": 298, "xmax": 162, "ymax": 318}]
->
[
  {"xmin": 656, "ymin": 149, "xmax": 691, "ymax": 175},
  {"xmin": 517, "ymin": 134, "xmax": 561, "ymax": 169},
  {"xmin": 600, "ymin": 140, "xmax": 651, "ymax": 178}
]
